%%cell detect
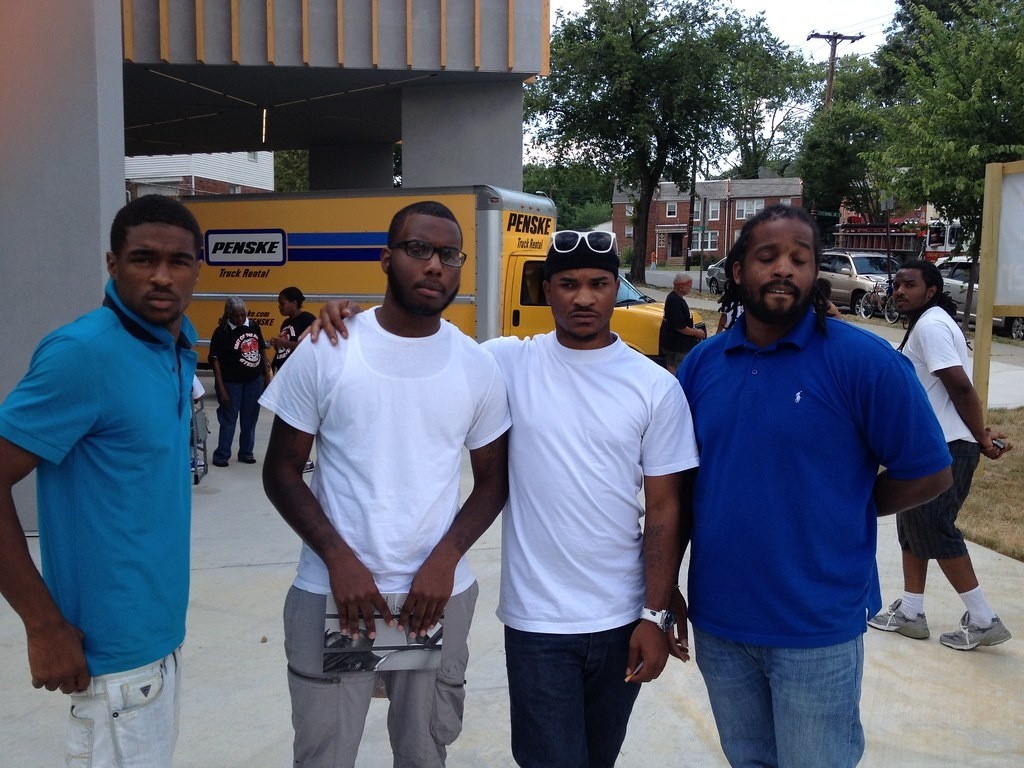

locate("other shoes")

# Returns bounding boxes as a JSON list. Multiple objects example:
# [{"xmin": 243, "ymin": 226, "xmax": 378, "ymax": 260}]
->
[
  {"xmin": 244, "ymin": 459, "xmax": 256, "ymax": 464},
  {"xmin": 213, "ymin": 461, "xmax": 228, "ymax": 467}
]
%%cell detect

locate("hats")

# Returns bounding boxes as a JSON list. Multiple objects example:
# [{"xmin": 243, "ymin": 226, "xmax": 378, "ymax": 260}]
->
[{"xmin": 544, "ymin": 228, "xmax": 620, "ymax": 280}]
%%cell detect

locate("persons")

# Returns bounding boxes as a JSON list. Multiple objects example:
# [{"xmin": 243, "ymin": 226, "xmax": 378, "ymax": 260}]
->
[
  {"xmin": 191, "ymin": 374, "xmax": 206, "ymax": 472},
  {"xmin": 826, "ymin": 298, "xmax": 843, "ymax": 319},
  {"xmin": 257, "ymin": 199, "xmax": 513, "ymax": 766},
  {"xmin": 663, "ymin": 203, "xmax": 954, "ymax": 767},
  {"xmin": 266, "ymin": 286, "xmax": 317, "ymax": 473},
  {"xmin": 0, "ymin": 192, "xmax": 203, "ymax": 766},
  {"xmin": 715, "ymin": 297, "xmax": 745, "ymax": 334},
  {"xmin": 660, "ymin": 272, "xmax": 705, "ymax": 377},
  {"xmin": 297, "ymin": 230, "xmax": 700, "ymax": 768},
  {"xmin": 649, "ymin": 249, "xmax": 656, "ymax": 271},
  {"xmin": 210, "ymin": 295, "xmax": 269, "ymax": 467},
  {"xmin": 868, "ymin": 261, "xmax": 1014, "ymax": 651},
  {"xmin": 929, "ymin": 231, "xmax": 943, "ymax": 248}
]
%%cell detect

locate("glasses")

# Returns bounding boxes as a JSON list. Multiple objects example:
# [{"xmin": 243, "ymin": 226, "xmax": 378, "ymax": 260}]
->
[
  {"xmin": 390, "ymin": 240, "xmax": 467, "ymax": 267},
  {"xmin": 551, "ymin": 230, "xmax": 619, "ymax": 255}
]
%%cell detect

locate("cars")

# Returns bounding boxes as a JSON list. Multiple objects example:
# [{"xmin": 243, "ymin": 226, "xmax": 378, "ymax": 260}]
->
[
  {"xmin": 706, "ymin": 258, "xmax": 727, "ymax": 296},
  {"xmin": 821, "ymin": 250, "xmax": 900, "ymax": 318},
  {"xmin": 934, "ymin": 255, "xmax": 1024, "ymax": 341}
]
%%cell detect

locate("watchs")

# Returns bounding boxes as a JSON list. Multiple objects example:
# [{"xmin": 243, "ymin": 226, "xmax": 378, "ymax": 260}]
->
[{"xmin": 639, "ymin": 606, "xmax": 675, "ymax": 633}]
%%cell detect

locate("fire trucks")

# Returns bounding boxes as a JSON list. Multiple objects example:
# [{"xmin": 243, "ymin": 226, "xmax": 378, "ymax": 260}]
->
[{"xmin": 844, "ymin": 217, "xmax": 976, "ymax": 261}]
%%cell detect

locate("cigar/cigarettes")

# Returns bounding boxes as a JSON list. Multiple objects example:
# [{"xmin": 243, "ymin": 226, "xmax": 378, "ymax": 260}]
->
[{"xmin": 625, "ymin": 661, "xmax": 643, "ymax": 682}]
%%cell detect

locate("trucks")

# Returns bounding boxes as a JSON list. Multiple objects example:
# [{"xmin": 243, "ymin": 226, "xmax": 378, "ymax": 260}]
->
[{"xmin": 178, "ymin": 182, "xmax": 705, "ymax": 372}]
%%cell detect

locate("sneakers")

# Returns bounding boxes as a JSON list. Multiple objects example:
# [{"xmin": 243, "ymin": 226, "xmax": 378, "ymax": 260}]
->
[
  {"xmin": 940, "ymin": 610, "xmax": 1012, "ymax": 650},
  {"xmin": 867, "ymin": 598, "xmax": 930, "ymax": 639}
]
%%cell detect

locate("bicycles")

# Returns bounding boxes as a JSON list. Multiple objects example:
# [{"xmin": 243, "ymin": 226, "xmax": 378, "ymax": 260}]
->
[{"xmin": 861, "ymin": 275, "xmax": 899, "ymax": 324}]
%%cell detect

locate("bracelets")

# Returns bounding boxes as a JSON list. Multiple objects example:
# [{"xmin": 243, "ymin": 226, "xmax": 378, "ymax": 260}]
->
[
  {"xmin": 689, "ymin": 327, "xmax": 698, "ymax": 336},
  {"xmin": 674, "ymin": 585, "xmax": 680, "ymax": 592}
]
